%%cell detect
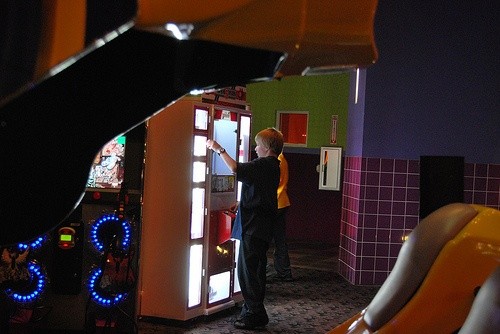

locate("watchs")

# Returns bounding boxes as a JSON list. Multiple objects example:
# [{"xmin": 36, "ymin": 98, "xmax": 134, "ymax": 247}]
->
[{"xmin": 217, "ymin": 148, "xmax": 226, "ymax": 156}]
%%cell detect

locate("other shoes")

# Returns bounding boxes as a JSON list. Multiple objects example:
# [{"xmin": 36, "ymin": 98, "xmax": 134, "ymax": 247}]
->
[
  {"xmin": 265, "ymin": 264, "xmax": 277, "ymax": 278},
  {"xmin": 232, "ymin": 305, "xmax": 270, "ymax": 328},
  {"xmin": 275, "ymin": 268, "xmax": 294, "ymax": 281}
]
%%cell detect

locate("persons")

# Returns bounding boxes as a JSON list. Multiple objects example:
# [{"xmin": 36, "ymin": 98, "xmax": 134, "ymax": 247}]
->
[
  {"xmin": 267, "ymin": 149, "xmax": 294, "ymax": 282},
  {"xmin": 204, "ymin": 128, "xmax": 284, "ymax": 329}
]
